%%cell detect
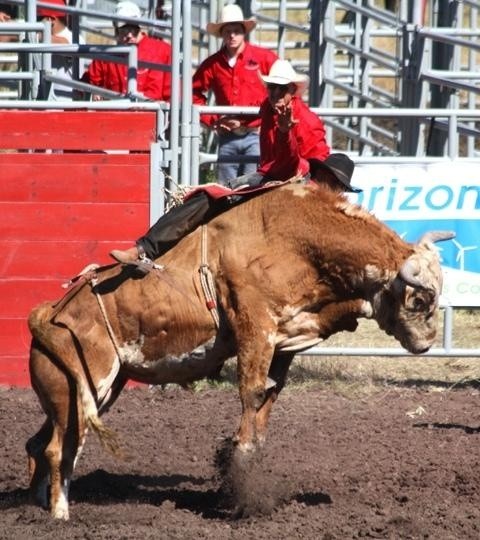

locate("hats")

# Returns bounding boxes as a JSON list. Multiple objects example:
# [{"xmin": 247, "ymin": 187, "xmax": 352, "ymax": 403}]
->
[
  {"xmin": 112, "ymin": 2, "xmax": 143, "ymax": 29},
  {"xmin": 308, "ymin": 153, "xmax": 364, "ymax": 192},
  {"xmin": 206, "ymin": 4, "xmax": 257, "ymax": 36},
  {"xmin": 36, "ymin": 0, "xmax": 67, "ymax": 19},
  {"xmin": 256, "ymin": 60, "xmax": 310, "ymax": 97}
]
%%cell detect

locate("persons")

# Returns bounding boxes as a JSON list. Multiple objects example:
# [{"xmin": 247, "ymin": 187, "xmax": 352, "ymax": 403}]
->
[
  {"xmin": 228, "ymin": 58, "xmax": 331, "ymax": 189},
  {"xmin": 192, "ymin": 4, "xmax": 280, "ymax": 188},
  {"xmin": 79, "ymin": 1, "xmax": 171, "ymax": 155},
  {"xmin": 108, "ymin": 153, "xmax": 363, "ymax": 276},
  {"xmin": 0, "ymin": 0, "xmax": 87, "ymax": 152}
]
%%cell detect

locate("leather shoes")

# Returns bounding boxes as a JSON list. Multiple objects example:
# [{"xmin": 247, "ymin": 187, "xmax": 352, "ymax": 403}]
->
[{"xmin": 110, "ymin": 245, "xmax": 154, "ymax": 273}]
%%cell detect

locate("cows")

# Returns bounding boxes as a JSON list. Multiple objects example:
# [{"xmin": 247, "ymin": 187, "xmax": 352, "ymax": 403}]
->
[{"xmin": 24, "ymin": 179, "xmax": 457, "ymax": 523}]
